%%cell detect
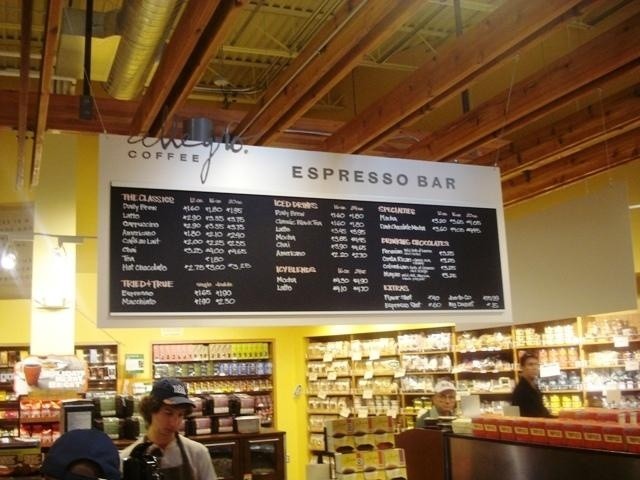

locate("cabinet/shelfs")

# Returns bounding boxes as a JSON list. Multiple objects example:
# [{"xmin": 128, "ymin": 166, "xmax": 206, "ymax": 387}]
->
[{"xmin": 0, "ymin": 311, "xmax": 639, "ymax": 480}]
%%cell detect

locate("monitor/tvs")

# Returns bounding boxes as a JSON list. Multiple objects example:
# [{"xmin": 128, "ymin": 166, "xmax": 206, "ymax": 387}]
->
[{"xmin": 59, "ymin": 398, "xmax": 95, "ymax": 435}]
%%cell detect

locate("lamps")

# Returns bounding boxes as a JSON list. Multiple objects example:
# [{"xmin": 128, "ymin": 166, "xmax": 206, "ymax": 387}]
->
[{"xmin": 1, "ymin": 242, "xmax": 17, "ymax": 270}]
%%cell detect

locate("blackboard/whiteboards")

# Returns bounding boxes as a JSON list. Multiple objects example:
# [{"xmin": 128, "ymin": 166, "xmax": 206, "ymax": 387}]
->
[{"xmin": 108, "ymin": 181, "xmax": 507, "ymax": 318}]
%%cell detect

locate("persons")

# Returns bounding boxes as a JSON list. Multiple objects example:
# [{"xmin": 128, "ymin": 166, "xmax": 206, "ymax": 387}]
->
[
  {"xmin": 118, "ymin": 376, "xmax": 218, "ymax": 479},
  {"xmin": 414, "ymin": 380, "xmax": 461, "ymax": 428},
  {"xmin": 511, "ymin": 353, "xmax": 559, "ymax": 418}
]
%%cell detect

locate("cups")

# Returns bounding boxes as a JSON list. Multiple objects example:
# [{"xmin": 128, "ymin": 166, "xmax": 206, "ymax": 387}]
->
[
  {"xmin": 424, "ymin": 417, "xmax": 438, "ymax": 426},
  {"xmin": 24, "ymin": 364, "xmax": 42, "ymax": 386}
]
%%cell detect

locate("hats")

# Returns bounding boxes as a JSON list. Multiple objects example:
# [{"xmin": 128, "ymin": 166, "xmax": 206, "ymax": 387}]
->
[
  {"xmin": 434, "ymin": 394, "xmax": 456, "ymax": 403},
  {"xmin": 433, "ymin": 380, "xmax": 457, "ymax": 395},
  {"xmin": 151, "ymin": 378, "xmax": 197, "ymax": 409}
]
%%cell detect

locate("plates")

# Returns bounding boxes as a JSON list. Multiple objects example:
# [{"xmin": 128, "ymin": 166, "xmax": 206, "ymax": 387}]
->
[{"xmin": 422, "ymin": 426, "xmax": 442, "ymax": 430}]
